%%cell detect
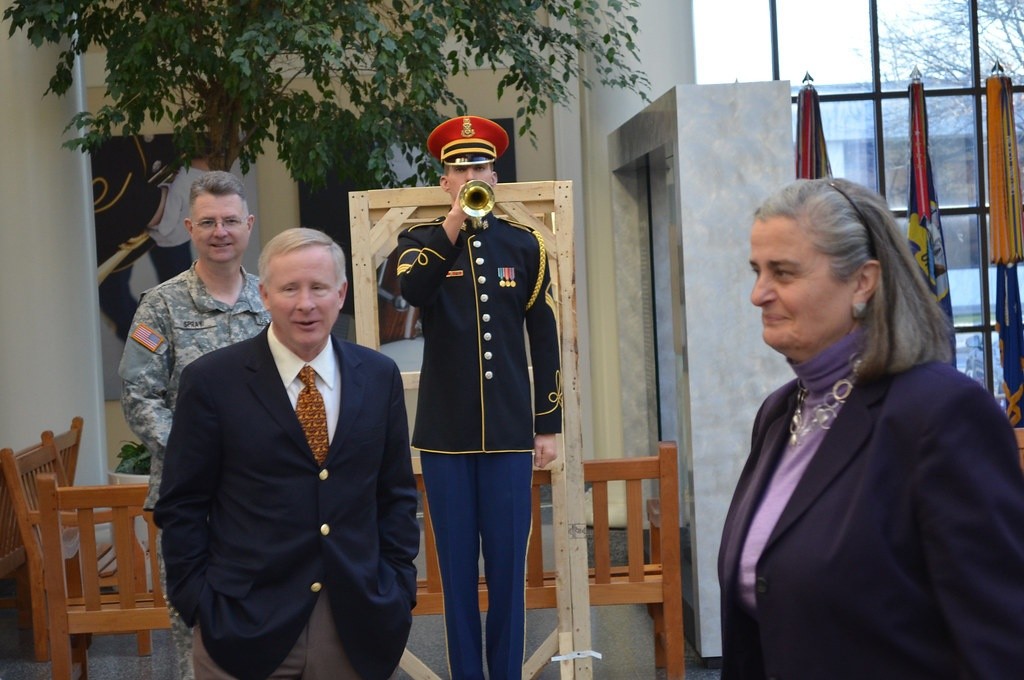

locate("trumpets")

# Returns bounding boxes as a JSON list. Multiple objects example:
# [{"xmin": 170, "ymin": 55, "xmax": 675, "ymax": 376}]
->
[{"xmin": 458, "ymin": 179, "xmax": 496, "ymax": 218}]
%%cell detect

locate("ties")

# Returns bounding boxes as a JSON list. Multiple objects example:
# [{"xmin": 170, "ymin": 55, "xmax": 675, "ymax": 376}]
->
[{"xmin": 295, "ymin": 366, "xmax": 330, "ymax": 466}]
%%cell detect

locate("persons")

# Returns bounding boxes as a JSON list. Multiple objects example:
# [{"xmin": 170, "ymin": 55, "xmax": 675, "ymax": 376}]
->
[
  {"xmin": 154, "ymin": 227, "xmax": 420, "ymax": 680},
  {"xmin": 117, "ymin": 171, "xmax": 273, "ymax": 680},
  {"xmin": 716, "ymin": 178, "xmax": 1024, "ymax": 680},
  {"xmin": 398, "ymin": 115, "xmax": 564, "ymax": 680}
]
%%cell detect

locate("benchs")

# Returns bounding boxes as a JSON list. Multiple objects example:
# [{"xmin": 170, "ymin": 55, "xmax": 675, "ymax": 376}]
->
[
  {"xmin": 0, "ymin": 416, "xmax": 85, "ymax": 631},
  {"xmin": 36, "ymin": 442, "xmax": 686, "ymax": 680}
]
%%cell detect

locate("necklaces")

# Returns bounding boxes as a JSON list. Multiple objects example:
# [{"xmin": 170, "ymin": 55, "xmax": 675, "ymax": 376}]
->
[{"xmin": 790, "ymin": 358, "xmax": 862, "ymax": 446}]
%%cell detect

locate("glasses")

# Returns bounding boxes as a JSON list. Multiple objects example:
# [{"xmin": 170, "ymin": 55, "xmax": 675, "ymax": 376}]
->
[{"xmin": 190, "ymin": 219, "xmax": 250, "ymax": 230}]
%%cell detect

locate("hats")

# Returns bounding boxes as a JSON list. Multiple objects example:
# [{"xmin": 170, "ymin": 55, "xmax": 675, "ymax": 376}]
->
[{"xmin": 426, "ymin": 115, "xmax": 510, "ymax": 167}]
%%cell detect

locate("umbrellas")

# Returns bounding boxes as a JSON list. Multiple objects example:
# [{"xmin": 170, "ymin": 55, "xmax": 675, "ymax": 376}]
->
[
  {"xmin": 797, "ymin": 72, "xmax": 832, "ymax": 179},
  {"xmin": 986, "ymin": 61, "xmax": 1024, "ymax": 265},
  {"xmin": 908, "ymin": 65, "xmax": 958, "ymax": 366}
]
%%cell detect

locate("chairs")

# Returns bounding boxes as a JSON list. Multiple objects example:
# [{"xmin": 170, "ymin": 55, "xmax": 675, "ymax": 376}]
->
[{"xmin": 0, "ymin": 430, "xmax": 156, "ymax": 663}]
